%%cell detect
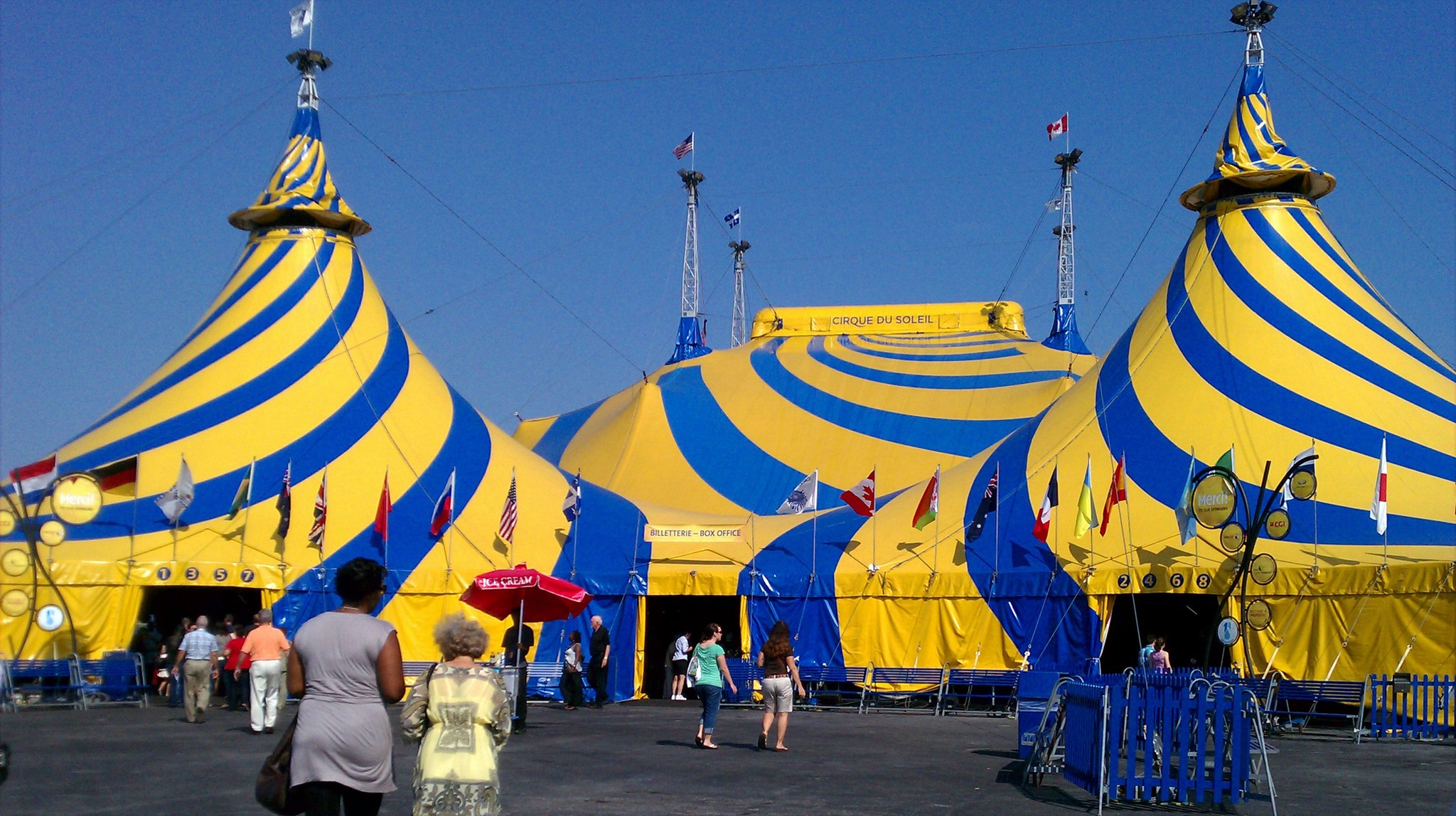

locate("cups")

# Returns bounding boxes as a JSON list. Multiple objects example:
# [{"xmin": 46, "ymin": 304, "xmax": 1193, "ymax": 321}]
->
[{"xmin": 150, "ymin": 615, "xmax": 155, "ymax": 622}]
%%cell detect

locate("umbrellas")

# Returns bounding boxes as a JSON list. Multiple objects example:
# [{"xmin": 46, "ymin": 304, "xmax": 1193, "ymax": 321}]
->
[{"xmin": 457, "ymin": 567, "xmax": 594, "ymax": 718}]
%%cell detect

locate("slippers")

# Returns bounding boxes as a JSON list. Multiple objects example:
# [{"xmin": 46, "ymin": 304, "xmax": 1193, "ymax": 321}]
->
[
  {"xmin": 775, "ymin": 747, "xmax": 787, "ymax": 751},
  {"xmin": 703, "ymin": 743, "xmax": 718, "ymax": 749},
  {"xmin": 695, "ymin": 736, "xmax": 703, "ymax": 746},
  {"xmin": 758, "ymin": 733, "xmax": 766, "ymax": 749}
]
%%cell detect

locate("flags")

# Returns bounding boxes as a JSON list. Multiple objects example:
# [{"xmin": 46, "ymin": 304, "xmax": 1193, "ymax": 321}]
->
[
  {"xmin": 1207, "ymin": 451, "xmax": 1232, "ymax": 484},
  {"xmin": 1032, "ymin": 457, "xmax": 1127, "ymax": 544},
  {"xmin": 1175, "ymin": 455, "xmax": 1197, "ymax": 544},
  {"xmin": 1046, "ymin": 194, "xmax": 1063, "ymax": 213},
  {"xmin": 1369, "ymin": 436, "xmax": 1387, "ymax": 535},
  {"xmin": 288, "ymin": 1, "xmax": 312, "ymax": 38},
  {"xmin": 910, "ymin": 468, "xmax": 937, "ymax": 530},
  {"xmin": 966, "ymin": 470, "xmax": 998, "ymax": 544},
  {"xmin": 777, "ymin": 470, "xmax": 815, "ymax": 515},
  {"xmin": 672, "ymin": 134, "xmax": 693, "ymax": 159},
  {"xmin": 10, "ymin": 457, "xmax": 194, "ymax": 525},
  {"xmin": 840, "ymin": 471, "xmax": 875, "ymax": 517},
  {"xmin": 724, "ymin": 209, "xmax": 739, "ymax": 229},
  {"xmin": 1046, "ymin": 114, "xmax": 1067, "ymax": 141},
  {"xmin": 563, "ymin": 473, "xmax": 581, "ymax": 522},
  {"xmin": 224, "ymin": 461, "xmax": 518, "ymax": 552},
  {"xmin": 1278, "ymin": 447, "xmax": 1315, "ymax": 512}
]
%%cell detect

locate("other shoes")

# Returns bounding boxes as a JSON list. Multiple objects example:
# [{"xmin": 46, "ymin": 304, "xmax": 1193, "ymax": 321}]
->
[
  {"xmin": 676, "ymin": 695, "xmax": 687, "ymax": 701},
  {"xmin": 250, "ymin": 728, "xmax": 263, "ymax": 735},
  {"xmin": 564, "ymin": 705, "xmax": 577, "ymax": 710},
  {"xmin": 514, "ymin": 726, "xmax": 525, "ymax": 734},
  {"xmin": 671, "ymin": 694, "xmax": 676, "ymax": 700},
  {"xmin": 220, "ymin": 703, "xmax": 229, "ymax": 708},
  {"xmin": 196, "ymin": 707, "xmax": 205, "ymax": 723},
  {"xmin": 242, "ymin": 703, "xmax": 248, "ymax": 711},
  {"xmin": 264, "ymin": 727, "xmax": 273, "ymax": 734},
  {"xmin": 589, "ymin": 703, "xmax": 602, "ymax": 710}
]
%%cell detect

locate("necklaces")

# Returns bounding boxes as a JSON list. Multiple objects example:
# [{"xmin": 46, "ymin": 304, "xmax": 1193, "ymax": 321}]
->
[{"xmin": 341, "ymin": 606, "xmax": 362, "ymax": 612}]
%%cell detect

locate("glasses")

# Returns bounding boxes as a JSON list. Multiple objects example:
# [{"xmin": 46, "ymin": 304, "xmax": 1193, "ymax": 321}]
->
[{"xmin": 714, "ymin": 631, "xmax": 722, "ymax": 634}]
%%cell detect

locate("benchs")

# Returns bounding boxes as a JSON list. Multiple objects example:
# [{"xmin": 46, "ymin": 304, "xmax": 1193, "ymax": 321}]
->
[
  {"xmin": 0, "ymin": 652, "xmax": 150, "ymax": 714},
  {"xmin": 717, "ymin": 662, "xmax": 1024, "ymax": 719},
  {"xmin": 401, "ymin": 659, "xmax": 596, "ymax": 704},
  {"xmin": 1200, "ymin": 673, "xmax": 1376, "ymax": 748}
]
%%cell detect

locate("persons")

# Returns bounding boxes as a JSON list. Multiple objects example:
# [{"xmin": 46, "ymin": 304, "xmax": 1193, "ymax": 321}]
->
[
  {"xmin": 1138, "ymin": 635, "xmax": 1173, "ymax": 674},
  {"xmin": 399, "ymin": 616, "xmax": 512, "ymax": 816},
  {"xmin": 502, "ymin": 608, "xmax": 612, "ymax": 733},
  {"xmin": 287, "ymin": 558, "xmax": 405, "ymax": 816},
  {"xmin": 662, "ymin": 620, "xmax": 806, "ymax": 751},
  {"xmin": 128, "ymin": 607, "xmax": 294, "ymax": 735}
]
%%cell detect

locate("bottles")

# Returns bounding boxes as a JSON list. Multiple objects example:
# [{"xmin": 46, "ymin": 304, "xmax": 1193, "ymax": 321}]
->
[
  {"xmin": 174, "ymin": 672, "xmax": 180, "ymax": 681},
  {"xmin": 500, "ymin": 652, "xmax": 505, "ymax": 667},
  {"xmin": 495, "ymin": 652, "xmax": 501, "ymax": 667},
  {"xmin": 490, "ymin": 652, "xmax": 495, "ymax": 666}
]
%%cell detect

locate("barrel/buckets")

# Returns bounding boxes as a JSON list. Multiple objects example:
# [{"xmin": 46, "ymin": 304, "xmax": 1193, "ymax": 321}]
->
[{"xmin": 27, "ymin": 696, "xmax": 41, "ymax": 704}]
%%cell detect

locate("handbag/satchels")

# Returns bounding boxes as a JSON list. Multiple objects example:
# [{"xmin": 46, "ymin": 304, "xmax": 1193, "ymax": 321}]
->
[
  {"xmin": 255, "ymin": 710, "xmax": 304, "ymax": 816},
  {"xmin": 689, "ymin": 642, "xmax": 701, "ymax": 676}
]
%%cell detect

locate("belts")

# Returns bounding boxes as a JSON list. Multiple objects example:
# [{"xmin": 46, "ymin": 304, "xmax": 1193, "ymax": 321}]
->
[
  {"xmin": 764, "ymin": 674, "xmax": 788, "ymax": 679},
  {"xmin": 592, "ymin": 652, "xmax": 605, "ymax": 656}
]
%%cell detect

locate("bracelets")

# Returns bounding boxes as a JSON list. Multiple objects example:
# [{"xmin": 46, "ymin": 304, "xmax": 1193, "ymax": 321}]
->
[
  {"xmin": 604, "ymin": 657, "xmax": 607, "ymax": 659},
  {"xmin": 572, "ymin": 665, "xmax": 576, "ymax": 667}
]
